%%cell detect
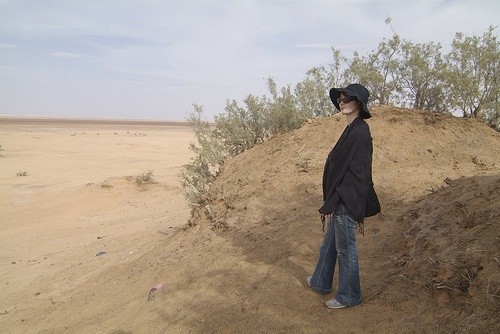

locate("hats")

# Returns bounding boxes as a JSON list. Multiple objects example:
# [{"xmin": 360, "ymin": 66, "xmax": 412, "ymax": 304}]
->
[{"xmin": 329, "ymin": 84, "xmax": 372, "ymax": 119}]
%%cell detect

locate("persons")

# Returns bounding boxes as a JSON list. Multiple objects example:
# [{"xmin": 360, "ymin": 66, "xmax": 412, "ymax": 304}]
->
[{"xmin": 305, "ymin": 83, "xmax": 373, "ymax": 308}]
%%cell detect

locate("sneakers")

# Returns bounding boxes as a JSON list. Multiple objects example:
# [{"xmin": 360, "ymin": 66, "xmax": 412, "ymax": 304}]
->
[
  {"xmin": 306, "ymin": 276, "xmax": 319, "ymax": 292},
  {"xmin": 325, "ymin": 297, "xmax": 360, "ymax": 308}
]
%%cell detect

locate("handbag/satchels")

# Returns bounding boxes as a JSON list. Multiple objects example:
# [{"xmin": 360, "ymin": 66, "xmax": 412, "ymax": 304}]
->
[{"xmin": 365, "ymin": 183, "xmax": 380, "ymax": 217}]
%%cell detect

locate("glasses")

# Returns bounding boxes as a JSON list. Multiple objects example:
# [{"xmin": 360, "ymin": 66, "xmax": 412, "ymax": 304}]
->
[{"xmin": 337, "ymin": 97, "xmax": 355, "ymax": 104}]
